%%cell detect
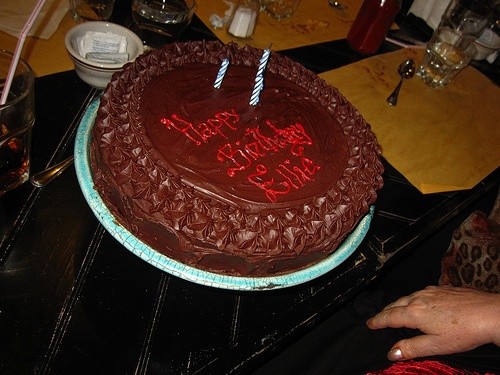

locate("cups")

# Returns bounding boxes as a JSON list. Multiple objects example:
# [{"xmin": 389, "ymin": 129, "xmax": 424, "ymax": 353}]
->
[
  {"xmin": 414, "ymin": 27, "xmax": 478, "ymax": 92},
  {"xmin": 474, "ymin": 28, "xmax": 500, "ymax": 61},
  {"xmin": 259, "ymin": 0, "xmax": 302, "ymax": 22},
  {"xmin": 65, "ymin": 19, "xmax": 144, "ymax": 88},
  {"xmin": 450, "ymin": 0, "xmax": 496, "ymax": 38},
  {"xmin": 132, "ymin": 0, "xmax": 196, "ymax": 51},
  {"xmin": 0, "ymin": 50, "xmax": 35, "ymax": 194}
]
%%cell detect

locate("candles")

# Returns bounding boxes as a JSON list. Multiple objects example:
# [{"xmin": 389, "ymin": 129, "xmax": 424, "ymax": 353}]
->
[
  {"xmin": 248, "ymin": 43, "xmax": 273, "ymax": 106},
  {"xmin": 214, "ymin": 58, "xmax": 230, "ymax": 89}
]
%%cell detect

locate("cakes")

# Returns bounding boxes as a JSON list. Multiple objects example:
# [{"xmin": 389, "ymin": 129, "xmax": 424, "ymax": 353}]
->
[{"xmin": 90, "ymin": 38, "xmax": 385, "ymax": 275}]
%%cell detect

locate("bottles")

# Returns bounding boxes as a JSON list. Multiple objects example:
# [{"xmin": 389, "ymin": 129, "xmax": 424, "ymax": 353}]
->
[
  {"xmin": 346, "ymin": 0, "xmax": 402, "ymax": 56},
  {"xmin": 226, "ymin": 0, "xmax": 262, "ymax": 41}
]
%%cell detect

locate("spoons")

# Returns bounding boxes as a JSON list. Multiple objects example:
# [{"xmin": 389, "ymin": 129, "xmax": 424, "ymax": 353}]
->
[
  {"xmin": 328, "ymin": 0, "xmax": 350, "ymax": 9},
  {"xmin": 386, "ymin": 59, "xmax": 415, "ymax": 107}
]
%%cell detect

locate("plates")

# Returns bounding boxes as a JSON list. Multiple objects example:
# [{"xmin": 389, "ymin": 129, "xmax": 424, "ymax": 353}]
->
[{"xmin": 73, "ymin": 94, "xmax": 375, "ymax": 291}]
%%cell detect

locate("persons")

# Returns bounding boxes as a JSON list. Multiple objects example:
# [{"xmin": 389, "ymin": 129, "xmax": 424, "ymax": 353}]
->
[{"xmin": 366, "ymin": 284, "xmax": 499, "ymax": 362}]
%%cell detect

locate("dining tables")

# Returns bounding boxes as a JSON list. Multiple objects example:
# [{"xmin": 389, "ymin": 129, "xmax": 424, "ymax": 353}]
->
[{"xmin": 0, "ymin": 0, "xmax": 500, "ymax": 375}]
